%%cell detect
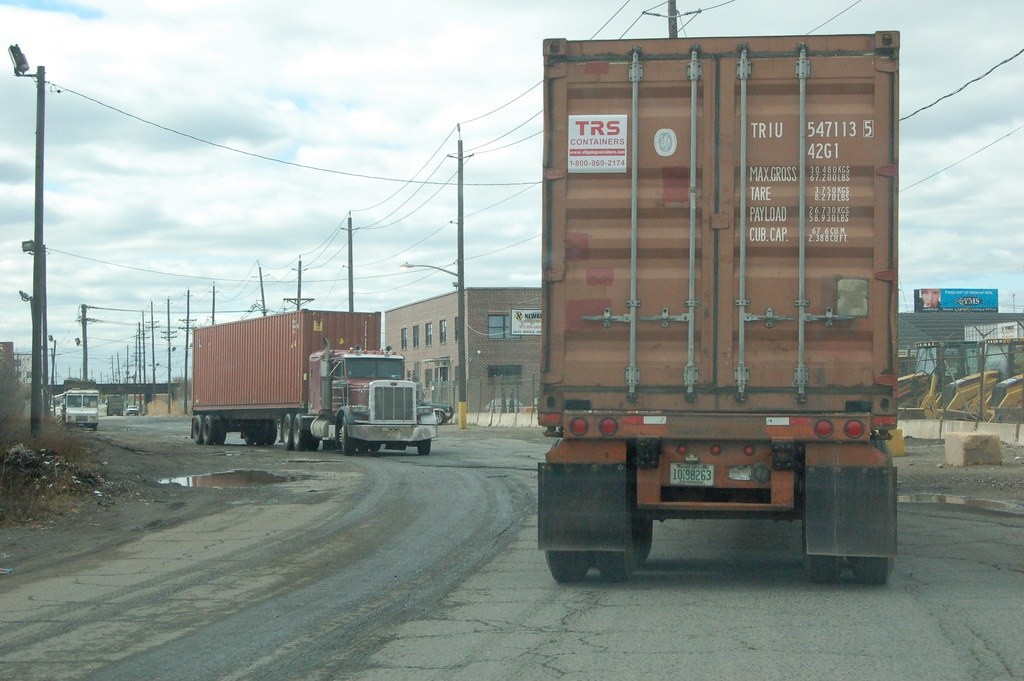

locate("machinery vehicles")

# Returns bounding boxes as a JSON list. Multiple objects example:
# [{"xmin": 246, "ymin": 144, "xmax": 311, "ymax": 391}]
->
[{"xmin": 899, "ymin": 334, "xmax": 1023, "ymax": 425}]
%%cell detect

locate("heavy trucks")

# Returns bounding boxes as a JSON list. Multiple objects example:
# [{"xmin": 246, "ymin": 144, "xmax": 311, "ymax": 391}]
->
[
  {"xmin": 533, "ymin": 30, "xmax": 901, "ymax": 588},
  {"xmin": 191, "ymin": 308, "xmax": 437, "ymax": 457}
]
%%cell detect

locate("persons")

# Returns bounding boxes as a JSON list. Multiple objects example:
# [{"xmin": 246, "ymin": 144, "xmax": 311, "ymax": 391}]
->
[{"xmin": 917, "ymin": 289, "xmax": 940, "ymax": 310}]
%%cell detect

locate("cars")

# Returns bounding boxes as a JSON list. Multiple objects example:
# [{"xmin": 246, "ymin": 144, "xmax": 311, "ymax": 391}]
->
[{"xmin": 484, "ymin": 397, "xmax": 526, "ymax": 413}]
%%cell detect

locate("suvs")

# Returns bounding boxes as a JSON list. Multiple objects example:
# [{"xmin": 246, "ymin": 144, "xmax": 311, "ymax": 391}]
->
[{"xmin": 415, "ymin": 383, "xmax": 452, "ymax": 426}]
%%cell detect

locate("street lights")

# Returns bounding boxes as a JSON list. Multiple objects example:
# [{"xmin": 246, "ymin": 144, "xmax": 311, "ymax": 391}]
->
[
  {"xmin": 47, "ymin": 334, "xmax": 57, "ymax": 397},
  {"xmin": 399, "ymin": 263, "xmax": 468, "ymax": 430},
  {"xmin": 168, "ymin": 346, "xmax": 177, "ymax": 415},
  {"xmin": 74, "ymin": 338, "xmax": 88, "ymax": 382},
  {"xmin": 155, "ymin": 363, "xmax": 171, "ymax": 414}
]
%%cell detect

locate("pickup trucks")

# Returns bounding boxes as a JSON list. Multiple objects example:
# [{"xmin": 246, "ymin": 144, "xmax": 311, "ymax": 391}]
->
[{"xmin": 125, "ymin": 406, "xmax": 138, "ymax": 416}]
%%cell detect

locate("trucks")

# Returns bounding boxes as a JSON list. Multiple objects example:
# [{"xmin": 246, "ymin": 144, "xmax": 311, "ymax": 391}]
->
[
  {"xmin": 51, "ymin": 390, "xmax": 99, "ymax": 432},
  {"xmin": 107, "ymin": 396, "xmax": 124, "ymax": 416}
]
